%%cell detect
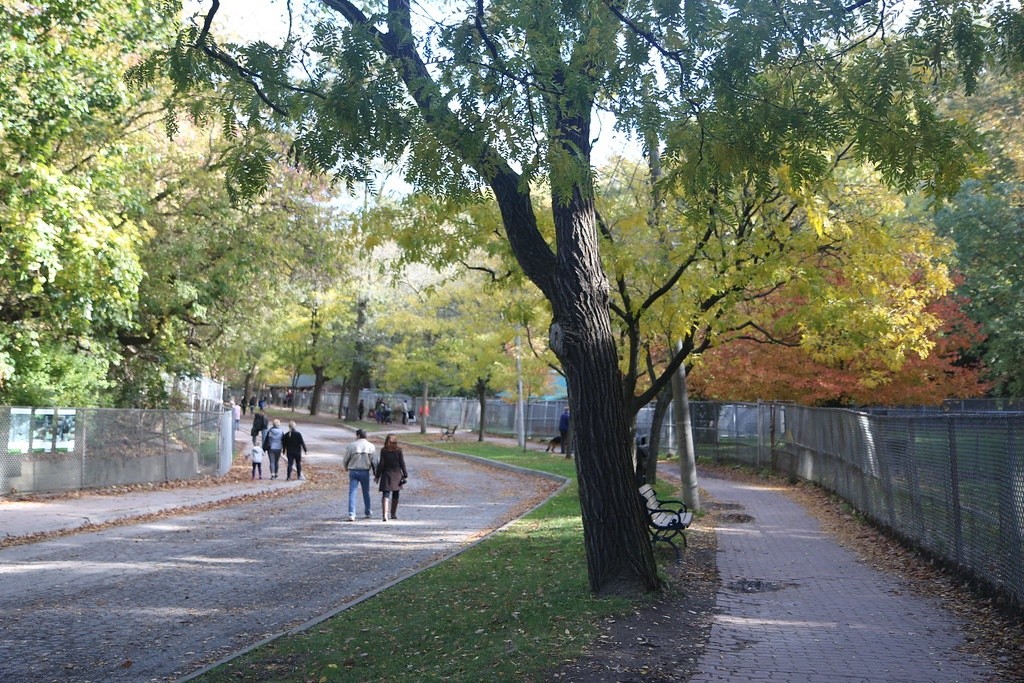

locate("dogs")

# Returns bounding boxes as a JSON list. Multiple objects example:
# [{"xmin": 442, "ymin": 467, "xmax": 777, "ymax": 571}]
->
[{"xmin": 545, "ymin": 436, "xmax": 561, "ymax": 453}]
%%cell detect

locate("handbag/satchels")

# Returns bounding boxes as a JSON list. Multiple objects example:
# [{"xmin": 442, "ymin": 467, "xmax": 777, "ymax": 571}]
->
[
  {"xmin": 263, "ymin": 431, "xmax": 270, "ymax": 451},
  {"xmin": 398, "ymin": 476, "xmax": 405, "ymax": 487}
]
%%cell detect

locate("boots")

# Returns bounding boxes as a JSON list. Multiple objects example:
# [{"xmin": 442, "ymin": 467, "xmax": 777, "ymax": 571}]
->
[
  {"xmin": 390, "ymin": 499, "xmax": 398, "ymax": 519},
  {"xmin": 382, "ymin": 497, "xmax": 388, "ymax": 521}
]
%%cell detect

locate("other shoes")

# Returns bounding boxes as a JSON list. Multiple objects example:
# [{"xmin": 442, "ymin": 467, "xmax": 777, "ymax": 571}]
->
[
  {"xmin": 298, "ymin": 476, "xmax": 302, "ymax": 480},
  {"xmin": 260, "ymin": 476, "xmax": 262, "ymax": 478},
  {"xmin": 366, "ymin": 510, "xmax": 372, "ymax": 518},
  {"xmin": 275, "ymin": 474, "xmax": 278, "ymax": 477},
  {"xmin": 270, "ymin": 476, "xmax": 274, "ymax": 479},
  {"xmin": 252, "ymin": 477, "xmax": 255, "ymax": 479},
  {"xmin": 349, "ymin": 516, "xmax": 354, "ymax": 520},
  {"xmin": 287, "ymin": 478, "xmax": 291, "ymax": 481}
]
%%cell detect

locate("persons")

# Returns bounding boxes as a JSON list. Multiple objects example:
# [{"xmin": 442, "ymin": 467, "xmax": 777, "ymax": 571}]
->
[
  {"xmin": 249, "ymin": 409, "xmax": 268, "ymax": 445},
  {"xmin": 419, "ymin": 401, "xmax": 428, "ymax": 416},
  {"xmin": 559, "ymin": 407, "xmax": 569, "ymax": 454},
  {"xmin": 286, "ymin": 390, "xmax": 293, "ymax": 408},
  {"xmin": 343, "ymin": 429, "xmax": 408, "ymax": 521},
  {"xmin": 249, "ymin": 398, "xmax": 254, "ymax": 414},
  {"xmin": 233, "ymin": 403, "xmax": 241, "ymax": 430},
  {"xmin": 375, "ymin": 397, "xmax": 392, "ymax": 425},
  {"xmin": 358, "ymin": 399, "xmax": 364, "ymax": 420},
  {"xmin": 245, "ymin": 419, "xmax": 307, "ymax": 481},
  {"xmin": 401, "ymin": 400, "xmax": 409, "ymax": 425},
  {"xmin": 241, "ymin": 397, "xmax": 247, "ymax": 414},
  {"xmin": 259, "ymin": 398, "xmax": 266, "ymax": 414}
]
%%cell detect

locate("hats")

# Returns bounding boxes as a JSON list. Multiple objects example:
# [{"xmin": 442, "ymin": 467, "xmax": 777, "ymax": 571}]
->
[{"xmin": 254, "ymin": 407, "xmax": 260, "ymax": 413}]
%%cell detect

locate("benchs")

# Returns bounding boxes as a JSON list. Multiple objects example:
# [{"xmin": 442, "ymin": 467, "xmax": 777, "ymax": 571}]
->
[
  {"xmin": 440, "ymin": 424, "xmax": 458, "ymax": 443},
  {"xmin": 639, "ymin": 483, "xmax": 693, "ymax": 565}
]
